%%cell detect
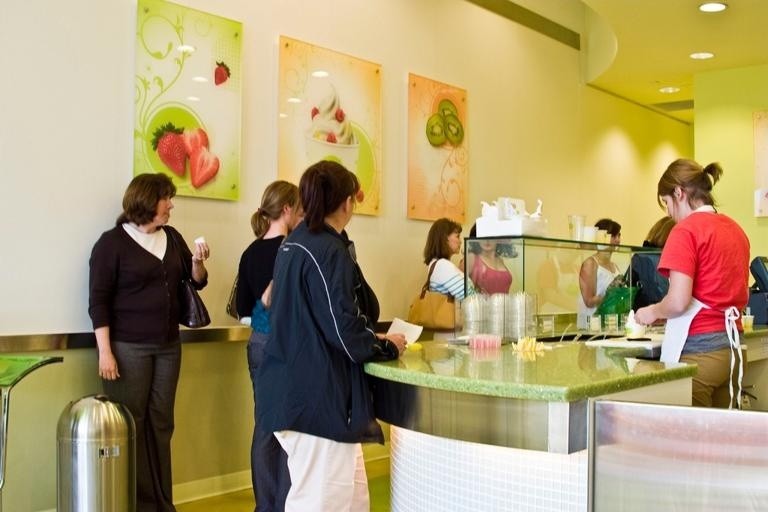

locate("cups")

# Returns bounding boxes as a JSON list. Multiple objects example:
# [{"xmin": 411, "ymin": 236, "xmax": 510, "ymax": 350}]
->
[
  {"xmin": 742, "ymin": 315, "xmax": 755, "ymax": 328},
  {"xmin": 568, "ymin": 214, "xmax": 611, "ymax": 244},
  {"xmin": 585, "ymin": 327, "xmax": 618, "ymax": 336},
  {"xmin": 624, "ymin": 326, "xmax": 647, "ymax": 338},
  {"xmin": 585, "ymin": 313, "xmax": 629, "ymax": 326}
]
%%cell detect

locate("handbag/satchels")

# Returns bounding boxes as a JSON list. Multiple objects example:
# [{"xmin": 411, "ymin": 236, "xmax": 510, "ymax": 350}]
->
[
  {"xmin": 595, "ymin": 286, "xmax": 641, "ymax": 326},
  {"xmin": 409, "ymin": 288, "xmax": 462, "ymax": 330},
  {"xmin": 177, "ymin": 280, "xmax": 211, "ymax": 329}
]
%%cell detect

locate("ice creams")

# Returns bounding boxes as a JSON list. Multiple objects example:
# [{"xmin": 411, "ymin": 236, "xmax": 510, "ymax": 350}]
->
[{"xmin": 307, "ymin": 83, "xmax": 355, "ymax": 147}]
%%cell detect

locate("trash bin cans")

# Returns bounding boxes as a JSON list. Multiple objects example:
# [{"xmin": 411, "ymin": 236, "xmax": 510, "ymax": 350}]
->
[{"xmin": 56, "ymin": 394, "xmax": 137, "ymax": 512}]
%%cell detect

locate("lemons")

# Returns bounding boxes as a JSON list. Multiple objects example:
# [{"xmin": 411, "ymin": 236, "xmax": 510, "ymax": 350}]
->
[{"xmin": 348, "ymin": 121, "xmax": 376, "ymax": 210}]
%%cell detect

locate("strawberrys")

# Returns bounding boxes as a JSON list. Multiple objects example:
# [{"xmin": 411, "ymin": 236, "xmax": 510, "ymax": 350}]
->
[
  {"xmin": 212, "ymin": 61, "xmax": 231, "ymax": 86},
  {"xmin": 152, "ymin": 122, "xmax": 221, "ymax": 190}
]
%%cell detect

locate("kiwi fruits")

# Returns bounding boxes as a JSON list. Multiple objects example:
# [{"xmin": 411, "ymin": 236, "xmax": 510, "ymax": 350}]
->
[{"xmin": 426, "ymin": 97, "xmax": 465, "ymax": 149}]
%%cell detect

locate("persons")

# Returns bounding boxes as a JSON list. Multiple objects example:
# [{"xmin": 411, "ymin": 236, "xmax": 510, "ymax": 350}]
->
[
  {"xmin": 234, "ymin": 179, "xmax": 303, "ymax": 511},
  {"xmin": 422, "ymin": 218, "xmax": 475, "ymax": 340},
  {"xmin": 458, "ymin": 219, "xmax": 519, "ymax": 294},
  {"xmin": 624, "ymin": 214, "xmax": 678, "ymax": 312},
  {"xmin": 632, "ymin": 159, "xmax": 750, "ymax": 409},
  {"xmin": 88, "ymin": 172, "xmax": 212, "ymax": 512},
  {"xmin": 257, "ymin": 158, "xmax": 409, "ymax": 511},
  {"xmin": 577, "ymin": 218, "xmax": 623, "ymax": 330}
]
